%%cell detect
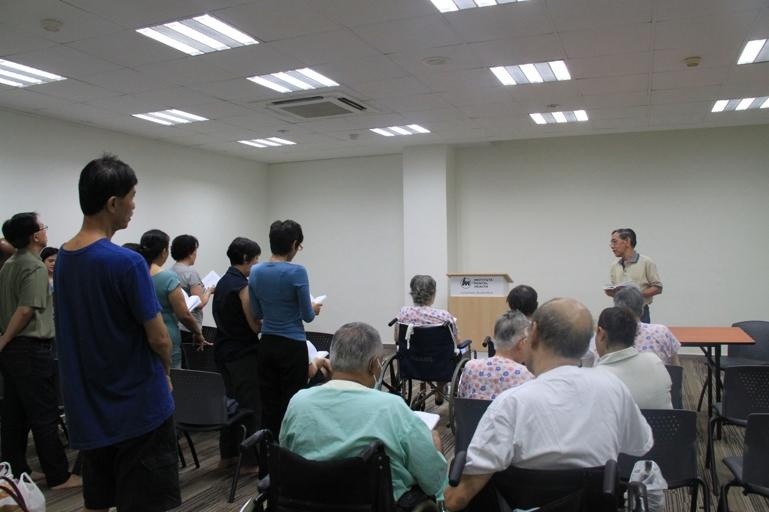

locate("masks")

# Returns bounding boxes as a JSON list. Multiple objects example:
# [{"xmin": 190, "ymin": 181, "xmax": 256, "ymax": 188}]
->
[{"xmin": 373, "ymin": 359, "xmax": 384, "ymax": 390}]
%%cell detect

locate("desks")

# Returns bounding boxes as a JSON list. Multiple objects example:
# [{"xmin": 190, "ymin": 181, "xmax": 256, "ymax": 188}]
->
[{"xmin": 669, "ymin": 325, "xmax": 755, "ymax": 403}]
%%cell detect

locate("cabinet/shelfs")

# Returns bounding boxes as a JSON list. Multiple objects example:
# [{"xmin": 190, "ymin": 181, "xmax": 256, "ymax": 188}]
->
[{"xmin": 448, "ymin": 273, "xmax": 514, "ymax": 353}]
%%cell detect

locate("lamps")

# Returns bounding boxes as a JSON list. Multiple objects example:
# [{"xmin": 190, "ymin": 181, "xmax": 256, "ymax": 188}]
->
[
  {"xmin": 245, "ymin": 67, "xmax": 340, "ymax": 93},
  {"xmin": 136, "ymin": 14, "xmax": 261, "ymax": 57},
  {"xmin": 369, "ymin": 123, "xmax": 432, "ymax": 137},
  {"xmin": 710, "ymin": 96, "xmax": 769, "ymax": 112},
  {"xmin": 490, "ymin": 59, "xmax": 571, "ymax": 85},
  {"xmin": 235, "ymin": 137, "xmax": 297, "ymax": 148},
  {"xmin": 738, "ymin": 37, "xmax": 769, "ymax": 65},
  {"xmin": 130, "ymin": 107, "xmax": 210, "ymax": 126},
  {"xmin": 0, "ymin": 59, "xmax": 66, "ymax": 89},
  {"xmin": 528, "ymin": 110, "xmax": 588, "ymax": 125},
  {"xmin": 430, "ymin": 0, "xmax": 526, "ymax": 14}
]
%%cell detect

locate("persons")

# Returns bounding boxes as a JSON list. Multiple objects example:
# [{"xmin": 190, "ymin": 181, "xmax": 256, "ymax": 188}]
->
[
  {"xmin": 279, "ymin": 322, "xmax": 449, "ymax": 503},
  {"xmin": 169, "ymin": 235, "xmax": 216, "ymax": 369},
  {"xmin": 140, "ymin": 230, "xmax": 214, "ymax": 368},
  {"xmin": 596, "ymin": 306, "xmax": 673, "ymax": 409},
  {"xmin": 0, "ymin": 238, "xmax": 17, "ymax": 270},
  {"xmin": 613, "ymin": 286, "xmax": 681, "ymax": 366},
  {"xmin": 307, "ymin": 340, "xmax": 331, "ymax": 388},
  {"xmin": 394, "ymin": 276, "xmax": 465, "ymax": 405},
  {"xmin": 0, "ymin": 213, "xmax": 83, "ymax": 491},
  {"xmin": 605, "ymin": 228, "xmax": 664, "ymax": 325},
  {"xmin": 53, "ymin": 157, "xmax": 183, "ymax": 511},
  {"xmin": 443, "ymin": 298, "xmax": 653, "ymax": 512},
  {"xmin": 40, "ymin": 247, "xmax": 59, "ymax": 288},
  {"xmin": 249, "ymin": 220, "xmax": 323, "ymax": 480},
  {"xmin": 458, "ymin": 310, "xmax": 537, "ymax": 401},
  {"xmin": 212, "ymin": 236, "xmax": 263, "ymax": 469},
  {"xmin": 506, "ymin": 285, "xmax": 538, "ymax": 323}
]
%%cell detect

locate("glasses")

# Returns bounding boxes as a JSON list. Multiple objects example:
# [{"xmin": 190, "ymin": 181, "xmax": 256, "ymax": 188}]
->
[
  {"xmin": 39, "ymin": 224, "xmax": 49, "ymax": 231},
  {"xmin": 295, "ymin": 242, "xmax": 304, "ymax": 250},
  {"xmin": 524, "ymin": 325, "xmax": 531, "ymax": 337}
]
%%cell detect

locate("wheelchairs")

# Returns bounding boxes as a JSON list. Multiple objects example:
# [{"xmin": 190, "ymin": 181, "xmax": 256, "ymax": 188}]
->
[
  {"xmin": 448, "ymin": 448, "xmax": 650, "ymax": 512},
  {"xmin": 239, "ymin": 428, "xmax": 438, "ymax": 512},
  {"xmin": 377, "ymin": 317, "xmax": 478, "ymax": 437}
]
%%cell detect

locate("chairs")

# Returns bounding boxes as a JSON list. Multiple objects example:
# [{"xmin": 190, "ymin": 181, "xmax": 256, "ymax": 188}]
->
[
  {"xmin": 200, "ymin": 325, "xmax": 216, "ymax": 336},
  {"xmin": 181, "ymin": 329, "xmax": 192, "ymax": 342},
  {"xmin": 619, "ymin": 409, "xmax": 711, "ymax": 512},
  {"xmin": 720, "ymin": 413, "xmax": 769, "ymax": 512},
  {"xmin": 184, "ymin": 344, "xmax": 220, "ymax": 370},
  {"xmin": 665, "ymin": 362, "xmax": 683, "ymax": 409},
  {"xmin": 305, "ymin": 330, "xmax": 334, "ymax": 354},
  {"xmin": 172, "ymin": 368, "xmax": 253, "ymax": 476},
  {"xmin": 706, "ymin": 321, "xmax": 769, "ymax": 466}
]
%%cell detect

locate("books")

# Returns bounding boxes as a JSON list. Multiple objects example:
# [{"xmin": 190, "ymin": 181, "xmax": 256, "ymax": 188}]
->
[
  {"xmin": 182, "ymin": 290, "xmax": 200, "ymax": 313},
  {"xmin": 309, "ymin": 294, "xmax": 326, "ymax": 306},
  {"xmin": 413, "ymin": 410, "xmax": 440, "ymax": 432},
  {"xmin": 201, "ymin": 270, "xmax": 220, "ymax": 290},
  {"xmin": 306, "ymin": 340, "xmax": 329, "ymax": 364},
  {"xmin": 603, "ymin": 285, "xmax": 624, "ymax": 297}
]
